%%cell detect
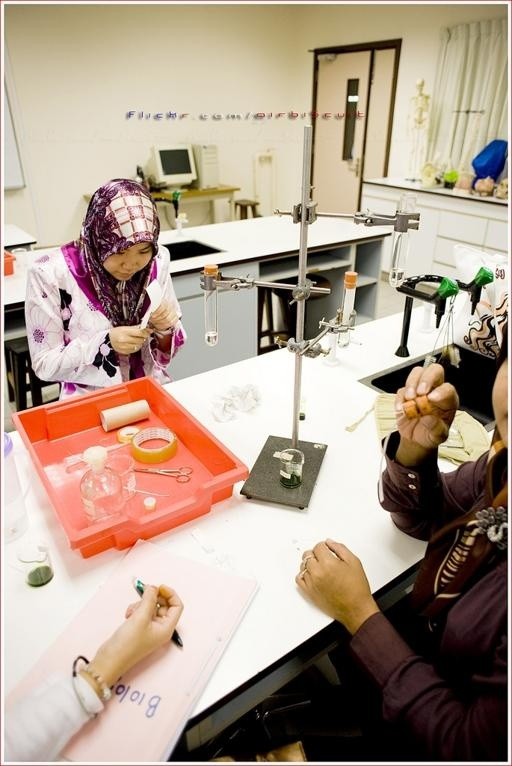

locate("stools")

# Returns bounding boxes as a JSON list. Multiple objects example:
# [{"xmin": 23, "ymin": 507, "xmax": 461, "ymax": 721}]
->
[
  {"xmin": 4, "ymin": 336, "xmax": 61, "ymax": 412},
  {"xmin": 258, "ymin": 273, "xmax": 331, "ymax": 354},
  {"xmin": 228, "ymin": 200, "xmax": 259, "ymax": 219}
]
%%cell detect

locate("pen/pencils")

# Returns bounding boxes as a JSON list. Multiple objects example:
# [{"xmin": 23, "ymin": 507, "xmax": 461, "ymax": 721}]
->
[{"xmin": 134, "ymin": 578, "xmax": 185, "ymax": 647}]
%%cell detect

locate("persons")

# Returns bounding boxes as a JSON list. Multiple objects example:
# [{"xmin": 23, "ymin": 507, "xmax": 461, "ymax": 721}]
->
[
  {"xmin": 295, "ymin": 358, "xmax": 509, "ymax": 762},
  {"xmin": 3, "ymin": 584, "xmax": 185, "ymax": 762},
  {"xmin": 25, "ymin": 179, "xmax": 187, "ymax": 402}
]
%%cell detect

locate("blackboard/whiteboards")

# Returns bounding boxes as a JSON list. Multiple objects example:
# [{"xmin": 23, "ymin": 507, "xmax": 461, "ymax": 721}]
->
[{"xmin": 3, "ymin": 40, "xmax": 26, "ymax": 191}]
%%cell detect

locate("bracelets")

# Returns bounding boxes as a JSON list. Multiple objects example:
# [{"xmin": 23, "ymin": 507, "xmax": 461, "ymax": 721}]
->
[
  {"xmin": 78, "ymin": 664, "xmax": 112, "ymax": 703},
  {"xmin": 71, "ymin": 656, "xmax": 98, "ymax": 718}
]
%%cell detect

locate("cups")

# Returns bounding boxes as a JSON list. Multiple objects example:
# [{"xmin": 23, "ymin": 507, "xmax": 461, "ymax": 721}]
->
[
  {"xmin": 279, "ymin": 448, "xmax": 305, "ymax": 489},
  {"xmin": 104, "ymin": 453, "xmax": 137, "ymax": 503},
  {"xmin": 14, "ymin": 541, "xmax": 54, "ymax": 587},
  {"xmin": 10, "ymin": 248, "xmax": 28, "ymax": 277},
  {"xmin": 4, "ymin": 431, "xmax": 29, "ymax": 542}
]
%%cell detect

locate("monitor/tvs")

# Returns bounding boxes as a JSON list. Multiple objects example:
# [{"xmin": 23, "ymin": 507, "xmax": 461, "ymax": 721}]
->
[{"xmin": 147, "ymin": 144, "xmax": 198, "ymax": 193}]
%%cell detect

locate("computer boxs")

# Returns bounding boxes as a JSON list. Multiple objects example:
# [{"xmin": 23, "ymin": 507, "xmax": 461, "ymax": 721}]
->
[{"xmin": 190, "ymin": 144, "xmax": 221, "ymax": 190}]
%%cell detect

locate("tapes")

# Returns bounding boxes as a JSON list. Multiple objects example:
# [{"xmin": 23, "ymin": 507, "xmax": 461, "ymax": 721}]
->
[
  {"xmin": 131, "ymin": 426, "xmax": 178, "ymax": 464},
  {"xmin": 117, "ymin": 426, "xmax": 143, "ymax": 443}
]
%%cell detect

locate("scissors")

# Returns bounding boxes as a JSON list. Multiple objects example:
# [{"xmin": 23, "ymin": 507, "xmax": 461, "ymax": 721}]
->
[{"xmin": 134, "ymin": 466, "xmax": 194, "ymax": 483}]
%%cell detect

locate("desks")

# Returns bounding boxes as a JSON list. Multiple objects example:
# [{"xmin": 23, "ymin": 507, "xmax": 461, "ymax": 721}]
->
[
  {"xmin": 361, "ymin": 179, "xmax": 510, "ymax": 292},
  {"xmin": 84, "ymin": 186, "xmax": 240, "ymax": 224},
  {"xmin": 4, "ymin": 224, "xmax": 37, "ymax": 252},
  {"xmin": 4, "ymin": 305, "xmax": 494, "ymax": 761},
  {"xmin": 4, "ymin": 215, "xmax": 393, "ymax": 383}
]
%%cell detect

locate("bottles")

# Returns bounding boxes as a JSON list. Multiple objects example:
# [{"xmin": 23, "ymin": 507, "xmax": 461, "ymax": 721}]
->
[
  {"xmin": 388, "ymin": 193, "xmax": 417, "ymax": 288},
  {"xmin": 202, "ymin": 263, "xmax": 219, "ymax": 346},
  {"xmin": 135, "ymin": 165, "xmax": 149, "ymax": 189},
  {"xmin": 336, "ymin": 271, "xmax": 358, "ymax": 349},
  {"xmin": 78, "ymin": 445, "xmax": 126, "ymax": 523}
]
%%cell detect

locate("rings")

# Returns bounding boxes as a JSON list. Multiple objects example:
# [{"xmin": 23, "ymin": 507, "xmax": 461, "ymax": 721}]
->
[
  {"xmin": 300, "ymin": 568, "xmax": 308, "ymax": 578},
  {"xmin": 134, "ymin": 346, "xmax": 138, "ymax": 351},
  {"xmin": 303, "ymin": 554, "xmax": 313, "ymax": 566}
]
